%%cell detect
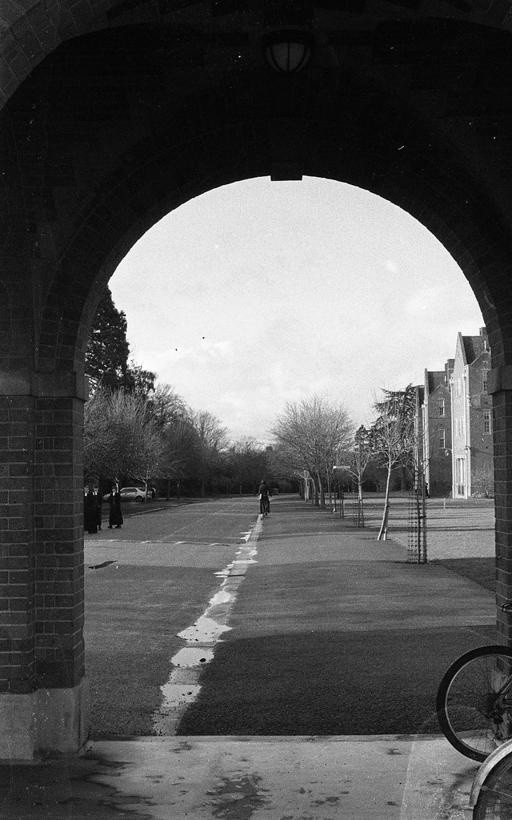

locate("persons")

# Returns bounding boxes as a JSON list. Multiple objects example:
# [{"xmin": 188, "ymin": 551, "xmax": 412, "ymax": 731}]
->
[
  {"xmin": 93, "ymin": 486, "xmax": 102, "ymax": 530},
  {"xmin": 84, "ymin": 481, "xmax": 97, "ymax": 534},
  {"xmin": 106, "ymin": 486, "xmax": 123, "ymax": 528},
  {"xmin": 255, "ymin": 480, "xmax": 272, "ymax": 520}
]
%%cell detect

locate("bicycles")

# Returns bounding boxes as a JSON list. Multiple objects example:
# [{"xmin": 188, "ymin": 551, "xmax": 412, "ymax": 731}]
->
[
  {"xmin": 435, "ymin": 602, "xmax": 512, "ymax": 819},
  {"xmin": 256, "ymin": 493, "xmax": 271, "ymax": 516}
]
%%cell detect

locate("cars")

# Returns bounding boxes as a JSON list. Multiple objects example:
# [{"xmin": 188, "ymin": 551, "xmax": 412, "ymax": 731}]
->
[{"xmin": 101, "ymin": 486, "xmax": 153, "ymax": 502}]
